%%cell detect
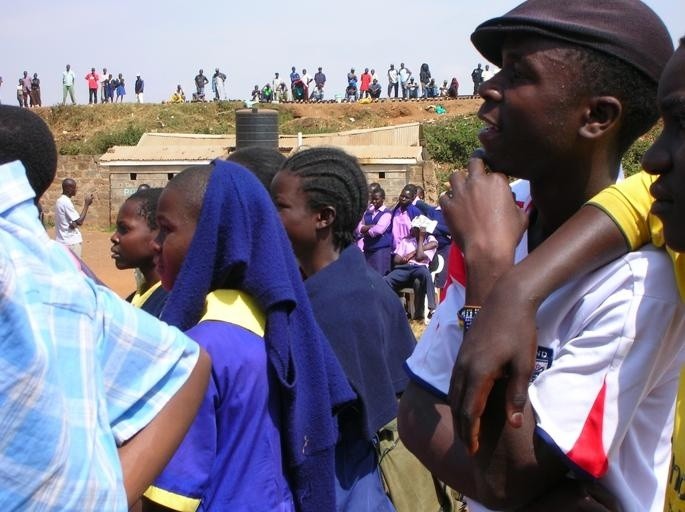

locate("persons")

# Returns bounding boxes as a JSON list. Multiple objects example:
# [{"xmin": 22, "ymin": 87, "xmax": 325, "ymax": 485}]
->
[
  {"xmin": 392, "ymin": 0, "xmax": 685, "ymax": 511},
  {"xmin": 112, "ymin": 186, "xmax": 169, "ymax": 316},
  {"xmin": 100, "ymin": 68, "xmax": 126, "ymax": 104},
  {"xmin": 85, "ymin": 68, "xmax": 99, "ymax": 104},
  {"xmin": 388, "ymin": 64, "xmax": 399, "ymax": 98},
  {"xmin": 481, "ymin": 65, "xmax": 494, "ymax": 83},
  {"xmin": 252, "ymin": 72, "xmax": 288, "ymax": 103},
  {"xmin": 290, "ymin": 66, "xmax": 326, "ymax": 102},
  {"xmin": 447, "ymin": 77, "xmax": 458, "ymax": 97},
  {"xmin": 406, "ymin": 77, "xmax": 419, "ymax": 98},
  {"xmin": 2, "ymin": 105, "xmax": 58, "ymax": 216},
  {"xmin": 439, "ymin": 80, "xmax": 448, "ymax": 97},
  {"xmin": 270, "ymin": 146, "xmax": 419, "ymax": 509},
  {"xmin": 398, "ymin": 63, "xmax": 411, "ymax": 98},
  {"xmin": 53, "ymin": 178, "xmax": 94, "ymax": 258},
  {"xmin": 17, "ymin": 71, "xmax": 41, "ymax": 108},
  {"xmin": 471, "ymin": 63, "xmax": 483, "ymax": 96},
  {"xmin": 174, "ymin": 84, "xmax": 185, "ymax": 102},
  {"xmin": 137, "ymin": 159, "xmax": 353, "ymax": 512},
  {"xmin": 2, "ymin": 160, "xmax": 213, "ymax": 511},
  {"xmin": 420, "ymin": 64, "xmax": 438, "ymax": 98},
  {"xmin": 226, "ymin": 146, "xmax": 286, "ymax": 199},
  {"xmin": 355, "ymin": 182, "xmax": 453, "ymax": 321},
  {"xmin": 192, "ymin": 69, "xmax": 209, "ymax": 102},
  {"xmin": 62, "ymin": 64, "xmax": 76, "ymax": 105},
  {"xmin": 212, "ymin": 68, "xmax": 226, "ymax": 100},
  {"xmin": 135, "ymin": 76, "xmax": 144, "ymax": 103},
  {"xmin": 346, "ymin": 67, "xmax": 381, "ymax": 99},
  {"xmin": 446, "ymin": 27, "xmax": 685, "ymax": 512}
]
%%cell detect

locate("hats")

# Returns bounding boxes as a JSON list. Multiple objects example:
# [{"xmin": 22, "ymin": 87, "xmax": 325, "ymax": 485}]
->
[{"xmin": 470, "ymin": 0, "xmax": 674, "ymax": 84}]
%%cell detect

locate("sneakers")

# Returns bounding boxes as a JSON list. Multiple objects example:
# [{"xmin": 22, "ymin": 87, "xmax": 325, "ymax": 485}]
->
[{"xmin": 427, "ymin": 312, "xmax": 434, "ymax": 319}]
